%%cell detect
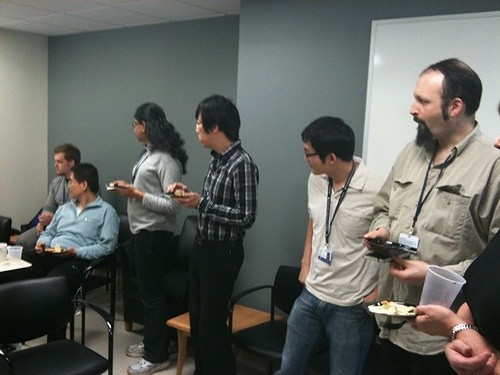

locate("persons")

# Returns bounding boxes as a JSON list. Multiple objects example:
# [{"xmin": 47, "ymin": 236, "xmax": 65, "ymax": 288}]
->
[
  {"xmin": 15, "ymin": 144, "xmax": 101, "ymax": 254},
  {"xmin": 165, "ymin": 96, "xmax": 259, "ymax": 375},
  {"xmin": 363, "ymin": 59, "xmax": 500, "ymax": 375},
  {"xmin": 109, "ymin": 102, "xmax": 189, "ymax": 375},
  {"xmin": 7, "ymin": 164, "xmax": 120, "ymax": 352},
  {"xmin": 275, "ymin": 115, "xmax": 379, "ymax": 375},
  {"xmin": 395, "ymin": 102, "xmax": 500, "ymax": 375}
]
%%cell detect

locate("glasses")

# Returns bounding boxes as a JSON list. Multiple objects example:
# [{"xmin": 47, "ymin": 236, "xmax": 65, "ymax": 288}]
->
[
  {"xmin": 304, "ymin": 150, "xmax": 318, "ymax": 158},
  {"xmin": 131, "ymin": 122, "xmax": 142, "ymax": 128}
]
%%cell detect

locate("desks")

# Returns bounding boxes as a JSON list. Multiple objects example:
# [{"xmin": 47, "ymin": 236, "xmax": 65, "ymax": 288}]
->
[{"xmin": 167, "ymin": 304, "xmax": 282, "ymax": 375}]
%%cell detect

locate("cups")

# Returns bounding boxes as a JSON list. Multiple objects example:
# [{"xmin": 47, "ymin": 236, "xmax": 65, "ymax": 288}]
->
[
  {"xmin": 7, "ymin": 247, "xmax": 23, "ymax": 266},
  {"xmin": 0, "ymin": 243, "xmax": 7, "ymax": 259},
  {"xmin": 419, "ymin": 265, "xmax": 467, "ymax": 311}
]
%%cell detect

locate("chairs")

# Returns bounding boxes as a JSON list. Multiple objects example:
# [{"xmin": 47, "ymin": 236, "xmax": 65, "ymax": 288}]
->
[
  {"xmin": 81, "ymin": 251, "xmax": 118, "ymax": 313},
  {"xmin": 123, "ymin": 215, "xmax": 199, "ymax": 341},
  {"xmin": 0, "ymin": 277, "xmax": 115, "ymax": 375},
  {"xmin": 229, "ymin": 266, "xmax": 305, "ymax": 375}
]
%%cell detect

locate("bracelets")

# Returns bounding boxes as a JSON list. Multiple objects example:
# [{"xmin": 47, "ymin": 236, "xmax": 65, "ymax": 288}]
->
[{"xmin": 449, "ymin": 323, "xmax": 480, "ymax": 342}]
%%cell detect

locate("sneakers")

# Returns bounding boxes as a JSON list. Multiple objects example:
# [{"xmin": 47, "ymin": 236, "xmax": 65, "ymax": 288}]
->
[
  {"xmin": 126, "ymin": 342, "xmax": 145, "ymax": 357},
  {"xmin": 127, "ymin": 360, "xmax": 171, "ymax": 375}
]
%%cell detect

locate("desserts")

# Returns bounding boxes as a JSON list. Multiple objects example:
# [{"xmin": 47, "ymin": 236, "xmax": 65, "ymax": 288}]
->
[
  {"xmin": 378, "ymin": 300, "xmax": 410, "ymax": 315},
  {"xmin": 54, "ymin": 243, "xmax": 61, "ymax": 252}
]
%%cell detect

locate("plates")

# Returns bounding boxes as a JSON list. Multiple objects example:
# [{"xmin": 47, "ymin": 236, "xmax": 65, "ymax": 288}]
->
[{"xmin": 368, "ymin": 301, "xmax": 417, "ymax": 317}]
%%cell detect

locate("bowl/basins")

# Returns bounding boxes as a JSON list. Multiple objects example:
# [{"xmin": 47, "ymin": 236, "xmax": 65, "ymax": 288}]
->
[{"xmin": 358, "ymin": 237, "xmax": 418, "ymax": 259}]
